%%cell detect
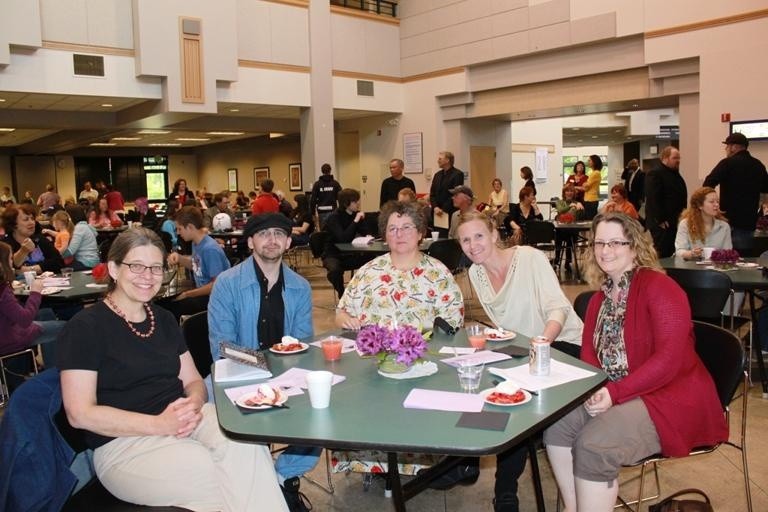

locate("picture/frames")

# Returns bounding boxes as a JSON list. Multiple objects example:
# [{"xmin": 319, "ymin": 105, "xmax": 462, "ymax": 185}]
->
[
  {"xmin": 289, "ymin": 163, "xmax": 302, "ymax": 191},
  {"xmin": 254, "ymin": 167, "xmax": 269, "ymax": 190},
  {"xmin": 228, "ymin": 169, "xmax": 238, "ymax": 192},
  {"xmin": 730, "ymin": 119, "xmax": 768, "ymax": 141}
]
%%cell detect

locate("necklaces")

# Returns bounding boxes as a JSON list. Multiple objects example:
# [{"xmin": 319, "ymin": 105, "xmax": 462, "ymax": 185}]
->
[{"xmin": 106, "ymin": 291, "xmax": 155, "ymax": 338}]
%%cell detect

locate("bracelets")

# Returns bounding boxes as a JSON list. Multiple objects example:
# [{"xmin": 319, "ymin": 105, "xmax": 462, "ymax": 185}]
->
[
  {"xmin": 692, "ymin": 249, "xmax": 698, "ymax": 258},
  {"xmin": 18, "ymin": 249, "xmax": 28, "ymax": 259}
]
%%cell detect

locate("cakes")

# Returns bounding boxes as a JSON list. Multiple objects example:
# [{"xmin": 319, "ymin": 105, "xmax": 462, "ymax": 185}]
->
[
  {"xmin": 243, "ymin": 384, "xmax": 290, "ymax": 409},
  {"xmin": 485, "ymin": 381, "xmax": 526, "ymax": 403},
  {"xmin": 272, "ymin": 335, "xmax": 303, "ymax": 351},
  {"xmin": 485, "ymin": 326, "xmax": 512, "ymax": 339}
]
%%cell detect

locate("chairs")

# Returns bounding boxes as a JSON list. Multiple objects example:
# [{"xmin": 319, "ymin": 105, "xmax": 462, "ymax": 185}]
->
[
  {"xmin": 185, "ymin": 311, "xmax": 213, "ymax": 378},
  {"xmin": 426, "ymin": 238, "xmax": 463, "ymax": 273},
  {"xmin": 309, "ymin": 231, "xmax": 330, "ymax": 258},
  {"xmin": 526, "ymin": 220, "xmax": 566, "ymax": 267},
  {"xmin": 573, "ymin": 290, "xmax": 598, "ymax": 321},
  {"xmin": 6, "ymin": 366, "xmax": 103, "ymax": 512},
  {"xmin": 0, "ymin": 340, "xmax": 38, "ymax": 409},
  {"xmin": 662, "ymin": 267, "xmax": 755, "ymax": 402},
  {"xmin": 617, "ymin": 318, "xmax": 754, "ymax": 511}
]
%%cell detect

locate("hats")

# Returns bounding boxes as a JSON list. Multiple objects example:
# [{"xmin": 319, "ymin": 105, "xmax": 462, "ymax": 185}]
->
[
  {"xmin": 720, "ymin": 131, "xmax": 751, "ymax": 149},
  {"xmin": 243, "ymin": 210, "xmax": 297, "ymax": 238}
]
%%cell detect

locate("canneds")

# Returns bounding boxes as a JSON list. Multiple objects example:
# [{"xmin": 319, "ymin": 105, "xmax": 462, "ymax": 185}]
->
[{"xmin": 529, "ymin": 336, "xmax": 550, "ymax": 378}]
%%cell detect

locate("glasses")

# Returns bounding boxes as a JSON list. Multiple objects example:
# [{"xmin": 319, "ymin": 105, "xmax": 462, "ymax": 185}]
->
[
  {"xmin": 256, "ymin": 228, "xmax": 287, "ymax": 239},
  {"xmin": 383, "ymin": 223, "xmax": 418, "ymax": 234},
  {"xmin": 594, "ymin": 239, "xmax": 633, "ymax": 249},
  {"xmin": 118, "ymin": 259, "xmax": 166, "ymax": 276}
]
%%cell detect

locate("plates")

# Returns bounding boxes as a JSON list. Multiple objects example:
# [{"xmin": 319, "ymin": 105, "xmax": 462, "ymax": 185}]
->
[
  {"xmin": 269, "ymin": 342, "xmax": 309, "ymax": 354},
  {"xmin": 736, "ymin": 263, "xmax": 759, "ymax": 268},
  {"xmin": 236, "ymin": 391, "xmax": 274, "ymax": 410},
  {"xmin": 480, "ymin": 388, "xmax": 532, "ymax": 406},
  {"xmin": 61, "ymin": 267, "xmax": 73, "ymax": 278},
  {"xmin": 41, "ymin": 286, "xmax": 62, "ymax": 295},
  {"xmin": 486, "ymin": 330, "xmax": 517, "ymax": 342}
]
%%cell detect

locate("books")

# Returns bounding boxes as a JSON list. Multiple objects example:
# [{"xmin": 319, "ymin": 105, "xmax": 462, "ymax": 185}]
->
[{"xmin": 215, "ymin": 341, "xmax": 272, "ymax": 383}]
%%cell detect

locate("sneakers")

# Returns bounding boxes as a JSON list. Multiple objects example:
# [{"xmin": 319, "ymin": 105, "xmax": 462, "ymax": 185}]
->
[
  {"xmin": 491, "ymin": 489, "xmax": 523, "ymax": 512},
  {"xmin": 277, "ymin": 473, "xmax": 317, "ymax": 512},
  {"xmin": 417, "ymin": 467, "xmax": 479, "ymax": 492}
]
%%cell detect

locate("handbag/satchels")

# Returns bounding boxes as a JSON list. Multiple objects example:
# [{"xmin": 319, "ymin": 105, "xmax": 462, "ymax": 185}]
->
[{"xmin": 645, "ymin": 487, "xmax": 713, "ymax": 511}]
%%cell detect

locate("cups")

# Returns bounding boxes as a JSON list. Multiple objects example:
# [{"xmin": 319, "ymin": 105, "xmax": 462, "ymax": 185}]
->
[
  {"xmin": 699, "ymin": 247, "xmax": 716, "ymax": 260},
  {"xmin": 306, "ymin": 371, "xmax": 333, "ymax": 408},
  {"xmin": 466, "ymin": 324, "xmax": 488, "ymax": 351},
  {"xmin": 319, "ymin": 335, "xmax": 344, "ymax": 362},
  {"xmin": 457, "ymin": 358, "xmax": 484, "ymax": 390},
  {"xmin": 24, "ymin": 272, "xmax": 37, "ymax": 285},
  {"xmin": 431, "ymin": 232, "xmax": 439, "ymax": 241}
]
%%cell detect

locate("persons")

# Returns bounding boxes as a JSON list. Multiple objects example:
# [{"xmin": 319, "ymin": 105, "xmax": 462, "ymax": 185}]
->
[
  {"xmin": 544, "ymin": 211, "xmax": 729, "ymax": 512},
  {"xmin": 674, "ymin": 187, "xmax": 744, "ymax": 316},
  {"xmin": 252, "ymin": 179, "xmax": 279, "ymax": 217},
  {"xmin": 448, "ymin": 185, "xmax": 482, "ymax": 239},
  {"xmin": 310, "ymin": 164, "xmax": 342, "ymax": 228},
  {"xmin": 62, "ymin": 205, "xmax": 100, "ymax": 270},
  {"xmin": 205, "ymin": 193, "xmax": 216, "ymax": 208},
  {"xmin": 330, "ymin": 200, "xmax": 465, "ymax": 475},
  {"xmin": 0, "ymin": 187, "xmax": 17, "ymax": 208},
  {"xmin": 601, "ymin": 183, "xmax": 638, "ymax": 220},
  {"xmin": 203, "ymin": 193, "xmax": 237, "ymax": 248},
  {"xmin": 271, "ymin": 192, "xmax": 290, "ymax": 218},
  {"xmin": 231, "ymin": 191, "xmax": 249, "ymax": 209},
  {"xmin": 78, "ymin": 198, "xmax": 88, "ymax": 216},
  {"xmin": 289, "ymin": 194, "xmax": 314, "ymax": 250},
  {"xmin": 204, "ymin": 212, "xmax": 323, "ymax": 512},
  {"xmin": 756, "ymin": 202, "xmax": 768, "ymax": 231},
  {"xmin": 79, "ymin": 181, "xmax": 99, "ymax": 204},
  {"xmin": 621, "ymin": 159, "xmax": 645, "ymax": 212},
  {"xmin": 165, "ymin": 179, "xmax": 195, "ymax": 217},
  {"xmin": 94, "ymin": 181, "xmax": 110, "ymax": 215},
  {"xmin": 104, "ymin": 184, "xmax": 125, "ymax": 221},
  {"xmin": 646, "ymin": 147, "xmax": 687, "ymax": 259},
  {"xmin": 25, "ymin": 192, "xmax": 36, "ymax": 208},
  {"xmin": 380, "ymin": 159, "xmax": 415, "ymax": 210},
  {"xmin": 195, "ymin": 195, "xmax": 209, "ymax": 208},
  {"xmin": 417, "ymin": 211, "xmax": 585, "ymax": 511},
  {"xmin": 20, "ymin": 199, "xmax": 32, "ymax": 208},
  {"xmin": 160, "ymin": 199, "xmax": 182, "ymax": 250},
  {"xmin": 756, "ymin": 250, "xmax": 768, "ymax": 345},
  {"xmin": 2, "ymin": 204, "xmax": 65, "ymax": 275},
  {"xmin": 485, "ymin": 179, "xmax": 510, "ymax": 229},
  {"xmin": 0, "ymin": 242, "xmax": 67, "ymax": 369},
  {"xmin": 430, "ymin": 152, "xmax": 464, "ymax": 238},
  {"xmin": 184, "ymin": 200, "xmax": 196, "ymax": 207},
  {"xmin": 276, "ymin": 191, "xmax": 292, "ymax": 212},
  {"xmin": 520, "ymin": 167, "xmax": 537, "ymax": 196},
  {"xmin": 583, "ymin": 155, "xmax": 603, "ymax": 220},
  {"xmin": 321, "ymin": 188, "xmax": 377, "ymax": 300},
  {"xmin": 153, "ymin": 207, "xmax": 231, "ymax": 326},
  {"xmin": 88, "ymin": 198, "xmax": 124, "ymax": 228},
  {"xmin": 37, "ymin": 184, "xmax": 63, "ymax": 210},
  {"xmin": 566, "ymin": 161, "xmax": 589, "ymax": 205},
  {"xmin": 5, "ymin": 200, "xmax": 13, "ymax": 208},
  {"xmin": 703, "ymin": 133, "xmax": 768, "ymax": 249},
  {"xmin": 41, "ymin": 199, "xmax": 65, "ymax": 221},
  {"xmin": 399, "ymin": 188, "xmax": 416, "ymax": 203},
  {"xmin": 42, "ymin": 210, "xmax": 74, "ymax": 258},
  {"xmin": 503, "ymin": 187, "xmax": 544, "ymax": 247},
  {"xmin": 249, "ymin": 191, "xmax": 259, "ymax": 208},
  {"xmin": 553, "ymin": 183, "xmax": 584, "ymax": 272},
  {"xmin": 124, "ymin": 197, "xmax": 157, "ymax": 233},
  {"xmin": 54, "ymin": 227, "xmax": 290, "ymax": 512}
]
